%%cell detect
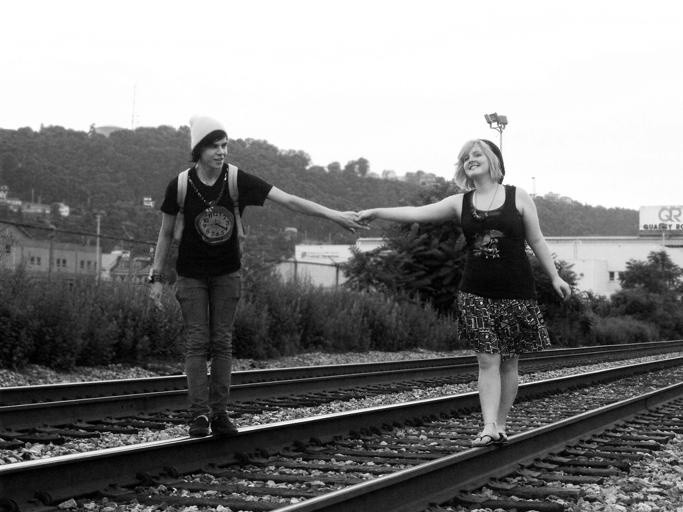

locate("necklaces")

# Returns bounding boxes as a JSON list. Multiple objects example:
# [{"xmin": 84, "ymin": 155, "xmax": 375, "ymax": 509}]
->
[
  {"xmin": 471, "ymin": 182, "xmax": 499, "ymax": 221},
  {"xmin": 187, "ymin": 168, "xmax": 228, "ymax": 213}
]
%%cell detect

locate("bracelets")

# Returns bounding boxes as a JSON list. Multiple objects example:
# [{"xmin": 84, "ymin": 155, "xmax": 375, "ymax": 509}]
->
[
  {"xmin": 147, "ymin": 275, "xmax": 166, "ymax": 284},
  {"xmin": 148, "ymin": 268, "xmax": 168, "ymax": 279}
]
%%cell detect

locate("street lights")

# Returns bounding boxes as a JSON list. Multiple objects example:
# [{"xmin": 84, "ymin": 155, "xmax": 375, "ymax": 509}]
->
[{"xmin": 484, "ymin": 112, "xmax": 508, "ymax": 151}]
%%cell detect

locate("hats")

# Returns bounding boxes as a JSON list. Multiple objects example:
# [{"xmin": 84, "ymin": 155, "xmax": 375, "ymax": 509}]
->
[
  {"xmin": 190, "ymin": 113, "xmax": 228, "ymax": 152},
  {"xmin": 481, "ymin": 139, "xmax": 505, "ymax": 184}
]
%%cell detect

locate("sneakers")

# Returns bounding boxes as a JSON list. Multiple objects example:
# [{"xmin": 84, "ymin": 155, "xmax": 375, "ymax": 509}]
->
[
  {"xmin": 189, "ymin": 417, "xmax": 209, "ymax": 437},
  {"xmin": 211, "ymin": 418, "xmax": 238, "ymax": 435}
]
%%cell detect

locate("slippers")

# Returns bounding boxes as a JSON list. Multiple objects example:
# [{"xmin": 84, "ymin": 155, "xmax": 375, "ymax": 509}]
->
[{"xmin": 471, "ymin": 433, "xmax": 509, "ymax": 447}]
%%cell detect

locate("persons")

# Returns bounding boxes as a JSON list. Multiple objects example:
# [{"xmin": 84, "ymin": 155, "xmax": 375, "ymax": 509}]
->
[
  {"xmin": 355, "ymin": 138, "xmax": 574, "ymax": 446},
  {"xmin": 146, "ymin": 112, "xmax": 369, "ymax": 437}
]
